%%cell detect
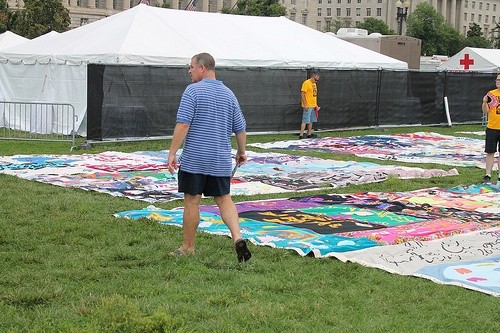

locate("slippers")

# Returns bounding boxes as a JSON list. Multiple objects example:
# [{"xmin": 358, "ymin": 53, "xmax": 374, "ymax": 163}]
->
[
  {"xmin": 167, "ymin": 250, "xmax": 186, "ymax": 258},
  {"xmin": 235, "ymin": 239, "xmax": 252, "ymax": 263}
]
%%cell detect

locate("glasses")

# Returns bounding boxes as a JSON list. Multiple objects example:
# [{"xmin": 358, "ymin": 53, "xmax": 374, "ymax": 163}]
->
[
  {"xmin": 313, "ymin": 77, "xmax": 320, "ymax": 82},
  {"xmin": 495, "ymin": 79, "xmax": 500, "ymax": 81}
]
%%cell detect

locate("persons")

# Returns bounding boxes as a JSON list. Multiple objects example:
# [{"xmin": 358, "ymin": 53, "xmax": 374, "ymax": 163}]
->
[
  {"xmin": 299, "ymin": 72, "xmax": 320, "ymax": 139},
  {"xmin": 167, "ymin": 52, "xmax": 252, "ymax": 264},
  {"xmin": 482, "ymin": 73, "xmax": 500, "ymax": 180}
]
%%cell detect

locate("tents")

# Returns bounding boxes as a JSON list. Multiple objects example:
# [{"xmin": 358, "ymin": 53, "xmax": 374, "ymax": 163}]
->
[{"xmin": 0, "ymin": 3, "xmax": 500, "ymax": 137}]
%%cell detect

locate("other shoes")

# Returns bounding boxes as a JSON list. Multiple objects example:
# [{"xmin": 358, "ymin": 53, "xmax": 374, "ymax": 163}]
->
[
  {"xmin": 298, "ymin": 135, "xmax": 306, "ymax": 140},
  {"xmin": 498, "ymin": 173, "xmax": 500, "ymax": 180},
  {"xmin": 308, "ymin": 133, "xmax": 317, "ymax": 139},
  {"xmin": 483, "ymin": 174, "xmax": 492, "ymax": 181}
]
